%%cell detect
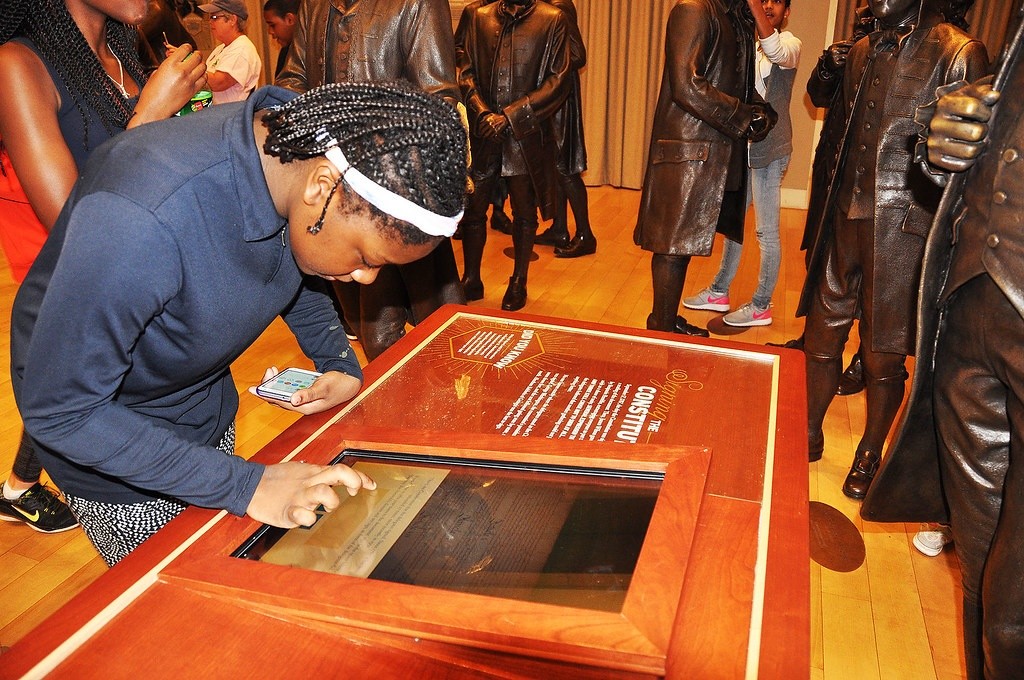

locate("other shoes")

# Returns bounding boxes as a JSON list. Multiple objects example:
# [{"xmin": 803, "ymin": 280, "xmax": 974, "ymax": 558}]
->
[{"xmin": 340, "ymin": 322, "xmax": 357, "ymax": 341}]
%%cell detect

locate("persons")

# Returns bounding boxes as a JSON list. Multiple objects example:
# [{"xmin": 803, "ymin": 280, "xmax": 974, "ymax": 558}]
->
[
  {"xmin": 450, "ymin": 1, "xmax": 597, "ymax": 310},
  {"xmin": 274, "ymin": 1, "xmax": 466, "ymax": 360},
  {"xmin": 0, "ymin": 0, "xmax": 301, "ymax": 530},
  {"xmin": 760, "ymin": 0, "xmax": 1023, "ymax": 680},
  {"xmin": 8, "ymin": 80, "xmax": 464, "ymax": 572},
  {"xmin": 632, "ymin": 0, "xmax": 778, "ymax": 338},
  {"xmin": 682, "ymin": 0, "xmax": 802, "ymax": 328}
]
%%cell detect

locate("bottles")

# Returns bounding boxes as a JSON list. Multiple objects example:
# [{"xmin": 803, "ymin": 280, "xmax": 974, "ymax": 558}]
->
[{"xmin": 170, "ymin": 51, "xmax": 212, "ymax": 116}]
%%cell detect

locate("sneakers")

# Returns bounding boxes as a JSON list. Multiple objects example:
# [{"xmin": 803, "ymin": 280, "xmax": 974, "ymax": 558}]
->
[
  {"xmin": 0, "ymin": 480, "xmax": 80, "ymax": 534},
  {"xmin": 722, "ymin": 301, "xmax": 772, "ymax": 326},
  {"xmin": 913, "ymin": 521, "xmax": 954, "ymax": 557},
  {"xmin": 681, "ymin": 286, "xmax": 730, "ymax": 312}
]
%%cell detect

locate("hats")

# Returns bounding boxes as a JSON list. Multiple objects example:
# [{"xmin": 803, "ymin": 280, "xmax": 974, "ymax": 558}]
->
[{"xmin": 197, "ymin": 0, "xmax": 248, "ymax": 22}]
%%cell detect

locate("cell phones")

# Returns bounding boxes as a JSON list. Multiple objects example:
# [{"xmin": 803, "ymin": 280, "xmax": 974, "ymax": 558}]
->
[{"xmin": 256, "ymin": 368, "xmax": 322, "ymax": 402}]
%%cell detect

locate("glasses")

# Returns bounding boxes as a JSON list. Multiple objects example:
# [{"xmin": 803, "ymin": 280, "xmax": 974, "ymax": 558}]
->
[{"xmin": 209, "ymin": 13, "xmax": 226, "ymax": 20}]
